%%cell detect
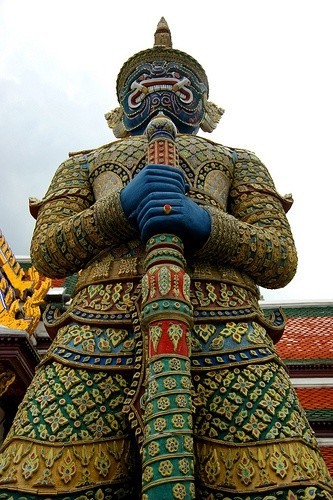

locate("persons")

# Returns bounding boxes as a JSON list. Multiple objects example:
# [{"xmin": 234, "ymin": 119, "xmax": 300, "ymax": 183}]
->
[{"xmin": 0, "ymin": 15, "xmax": 333, "ymax": 500}]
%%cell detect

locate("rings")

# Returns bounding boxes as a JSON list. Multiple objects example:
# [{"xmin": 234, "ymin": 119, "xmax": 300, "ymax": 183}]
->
[{"xmin": 163, "ymin": 204, "xmax": 171, "ymax": 214}]
